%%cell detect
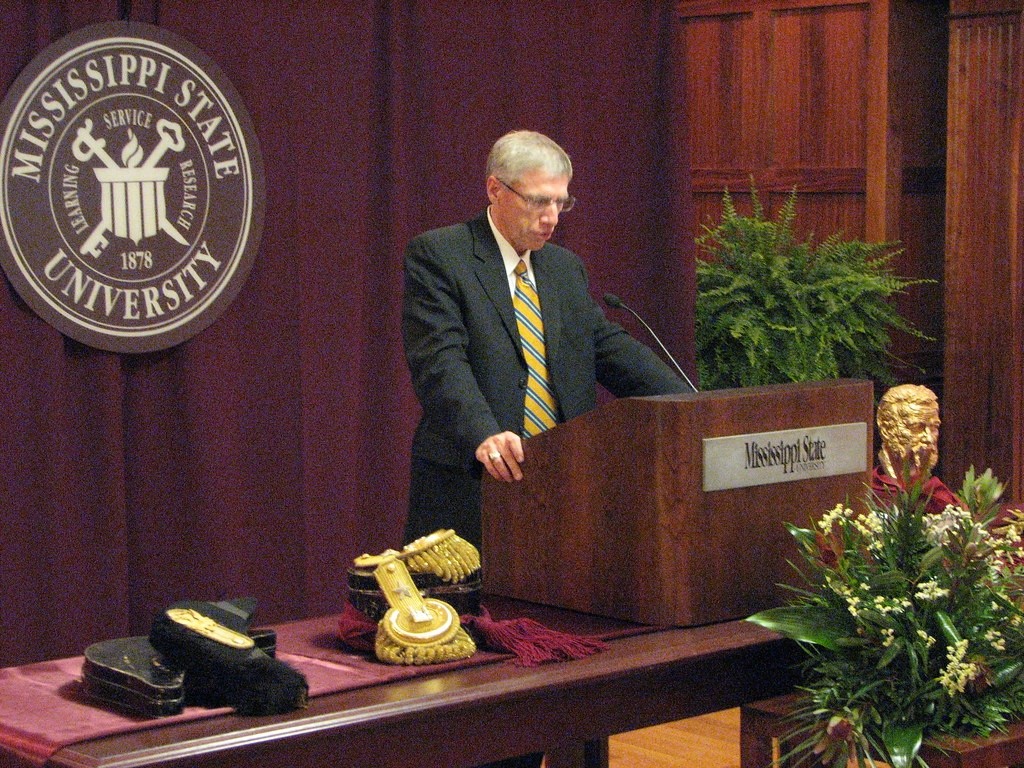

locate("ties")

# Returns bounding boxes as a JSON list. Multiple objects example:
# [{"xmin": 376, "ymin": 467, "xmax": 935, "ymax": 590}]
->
[{"xmin": 512, "ymin": 259, "xmax": 558, "ymax": 440}]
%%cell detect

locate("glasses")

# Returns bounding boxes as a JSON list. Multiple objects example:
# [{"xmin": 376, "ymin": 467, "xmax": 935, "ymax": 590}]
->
[{"xmin": 496, "ymin": 178, "xmax": 576, "ymax": 214}]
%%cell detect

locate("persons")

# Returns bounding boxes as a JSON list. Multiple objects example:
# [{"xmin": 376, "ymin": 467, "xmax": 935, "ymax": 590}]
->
[
  {"xmin": 873, "ymin": 382, "xmax": 973, "ymax": 522},
  {"xmin": 399, "ymin": 128, "xmax": 694, "ymax": 581}
]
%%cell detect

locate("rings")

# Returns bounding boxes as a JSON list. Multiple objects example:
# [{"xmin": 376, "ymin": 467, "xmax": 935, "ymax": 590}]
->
[{"xmin": 488, "ymin": 450, "xmax": 501, "ymax": 460}]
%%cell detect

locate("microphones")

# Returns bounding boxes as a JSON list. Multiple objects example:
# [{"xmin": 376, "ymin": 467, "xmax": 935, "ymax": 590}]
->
[{"xmin": 603, "ymin": 294, "xmax": 699, "ymax": 393}]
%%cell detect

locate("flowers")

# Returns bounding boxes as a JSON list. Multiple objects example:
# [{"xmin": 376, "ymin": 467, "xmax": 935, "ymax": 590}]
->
[{"xmin": 746, "ymin": 471, "xmax": 1024, "ymax": 768}]
